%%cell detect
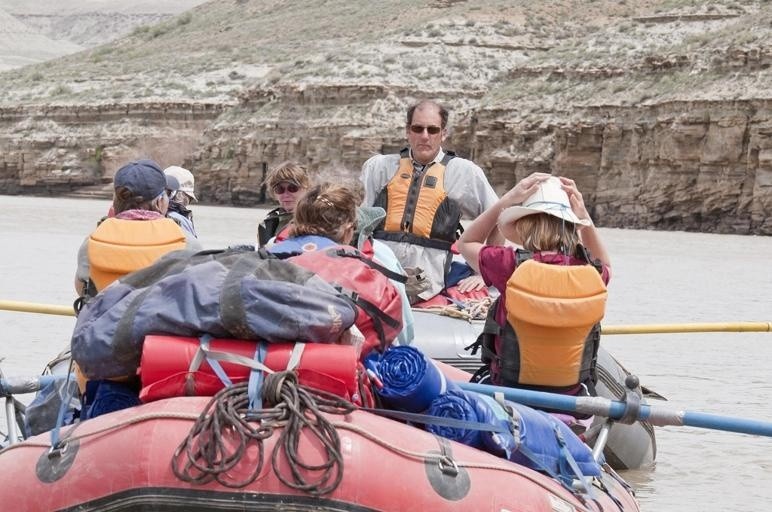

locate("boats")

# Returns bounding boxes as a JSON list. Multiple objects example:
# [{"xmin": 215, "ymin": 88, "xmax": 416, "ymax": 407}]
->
[
  {"xmin": 370, "ymin": 284, "xmax": 657, "ymax": 469},
  {"xmin": 0, "ymin": 354, "xmax": 646, "ymax": 512}
]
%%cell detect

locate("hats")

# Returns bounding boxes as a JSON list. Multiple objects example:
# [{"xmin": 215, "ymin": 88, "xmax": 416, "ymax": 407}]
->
[
  {"xmin": 497, "ymin": 176, "xmax": 591, "ymax": 245},
  {"xmin": 163, "ymin": 166, "xmax": 198, "ymax": 203},
  {"xmin": 114, "ymin": 160, "xmax": 179, "ymax": 203}
]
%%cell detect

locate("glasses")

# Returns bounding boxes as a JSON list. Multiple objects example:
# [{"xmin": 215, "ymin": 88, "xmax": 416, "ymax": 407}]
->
[
  {"xmin": 273, "ymin": 184, "xmax": 298, "ymax": 194},
  {"xmin": 407, "ymin": 124, "xmax": 441, "ymax": 134}
]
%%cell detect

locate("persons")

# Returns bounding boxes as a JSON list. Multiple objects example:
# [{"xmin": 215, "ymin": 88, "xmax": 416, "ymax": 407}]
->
[
  {"xmin": 259, "ymin": 160, "xmax": 308, "ymax": 244},
  {"xmin": 430, "ymin": 173, "xmax": 613, "ymax": 419},
  {"xmin": 358, "ymin": 101, "xmax": 506, "ymax": 306},
  {"xmin": 264, "ymin": 183, "xmax": 416, "ymax": 347},
  {"xmin": 165, "ymin": 163, "xmax": 201, "ymax": 237},
  {"xmin": 74, "ymin": 159, "xmax": 201, "ymax": 305}
]
